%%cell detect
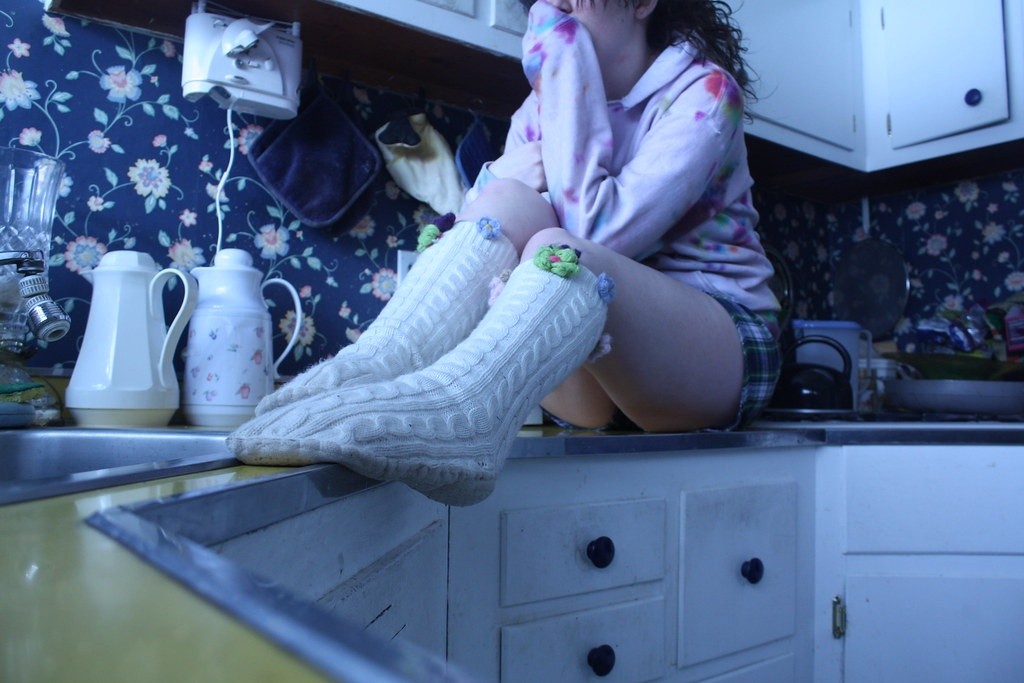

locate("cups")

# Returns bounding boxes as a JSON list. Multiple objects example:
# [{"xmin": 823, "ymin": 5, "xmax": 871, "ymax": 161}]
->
[
  {"xmin": 0, "ymin": 146, "xmax": 66, "ymax": 353},
  {"xmin": 858, "ymin": 357, "xmax": 898, "ymax": 414}
]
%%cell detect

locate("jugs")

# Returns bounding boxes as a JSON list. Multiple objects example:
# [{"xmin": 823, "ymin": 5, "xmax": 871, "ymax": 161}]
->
[
  {"xmin": 184, "ymin": 248, "xmax": 303, "ymax": 425},
  {"xmin": 64, "ymin": 250, "xmax": 198, "ymax": 429},
  {"xmin": 791, "ymin": 319, "xmax": 873, "ymax": 409}
]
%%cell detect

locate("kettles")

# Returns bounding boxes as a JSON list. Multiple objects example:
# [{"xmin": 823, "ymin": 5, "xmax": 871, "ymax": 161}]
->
[{"xmin": 770, "ymin": 333, "xmax": 856, "ymax": 409}]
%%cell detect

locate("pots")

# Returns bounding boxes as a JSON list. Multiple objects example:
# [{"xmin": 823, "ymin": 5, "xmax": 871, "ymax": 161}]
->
[{"xmin": 881, "ymin": 362, "xmax": 1024, "ymax": 421}]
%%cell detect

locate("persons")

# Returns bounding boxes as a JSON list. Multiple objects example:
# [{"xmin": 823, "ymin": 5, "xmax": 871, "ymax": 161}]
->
[{"xmin": 225, "ymin": 0, "xmax": 783, "ymax": 507}]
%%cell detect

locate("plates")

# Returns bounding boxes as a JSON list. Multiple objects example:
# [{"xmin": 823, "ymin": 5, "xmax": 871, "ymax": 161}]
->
[
  {"xmin": 763, "ymin": 409, "xmax": 854, "ymax": 421},
  {"xmin": 764, "ymin": 245, "xmax": 796, "ymax": 332}
]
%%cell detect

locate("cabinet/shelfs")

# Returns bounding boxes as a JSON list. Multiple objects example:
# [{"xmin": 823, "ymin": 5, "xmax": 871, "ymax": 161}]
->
[
  {"xmin": 212, "ymin": 441, "xmax": 1024, "ymax": 683},
  {"xmin": 43, "ymin": 0, "xmax": 1024, "ymax": 195}
]
